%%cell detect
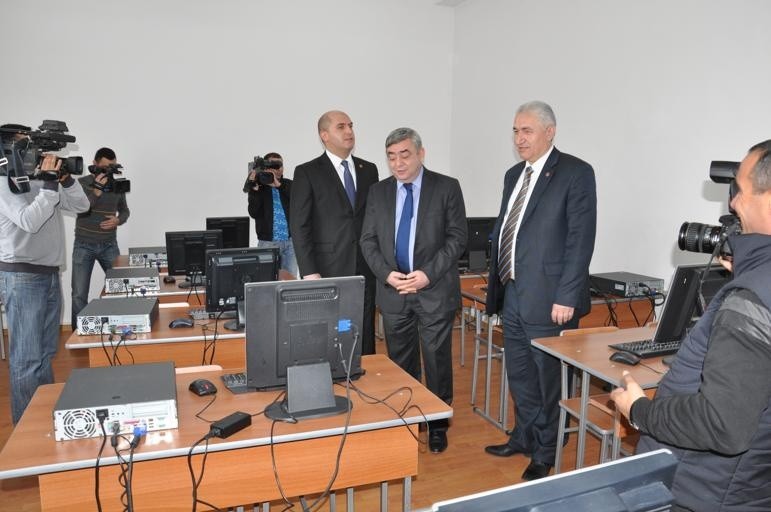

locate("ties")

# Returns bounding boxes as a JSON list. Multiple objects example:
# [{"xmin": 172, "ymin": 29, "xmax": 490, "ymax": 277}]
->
[
  {"xmin": 342, "ymin": 161, "xmax": 356, "ymax": 210},
  {"xmin": 394, "ymin": 183, "xmax": 412, "ymax": 273},
  {"xmin": 498, "ymin": 168, "xmax": 531, "ymax": 285}
]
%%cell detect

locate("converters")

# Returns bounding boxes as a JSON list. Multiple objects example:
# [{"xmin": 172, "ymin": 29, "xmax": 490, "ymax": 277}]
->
[{"xmin": 211, "ymin": 412, "xmax": 250, "ymax": 438}]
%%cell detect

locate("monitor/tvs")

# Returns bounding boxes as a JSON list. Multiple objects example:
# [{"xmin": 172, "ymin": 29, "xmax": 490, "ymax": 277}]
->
[
  {"xmin": 432, "ymin": 449, "xmax": 679, "ymax": 512},
  {"xmin": 244, "ymin": 275, "xmax": 365, "ymax": 420},
  {"xmin": 458, "ymin": 217, "xmax": 498, "ymax": 275},
  {"xmin": 651, "ymin": 264, "xmax": 733, "ymax": 367},
  {"xmin": 165, "ymin": 217, "xmax": 281, "ymax": 332}
]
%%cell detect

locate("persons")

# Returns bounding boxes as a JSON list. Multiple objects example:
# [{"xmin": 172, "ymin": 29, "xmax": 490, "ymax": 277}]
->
[
  {"xmin": 71, "ymin": 148, "xmax": 131, "ymax": 332},
  {"xmin": 289, "ymin": 111, "xmax": 379, "ymax": 355},
  {"xmin": 485, "ymin": 100, "xmax": 597, "ymax": 481},
  {"xmin": 0, "ymin": 124, "xmax": 91, "ymax": 427},
  {"xmin": 360, "ymin": 128, "xmax": 468, "ymax": 452},
  {"xmin": 608, "ymin": 139, "xmax": 771, "ymax": 512},
  {"xmin": 248, "ymin": 152, "xmax": 297, "ymax": 279}
]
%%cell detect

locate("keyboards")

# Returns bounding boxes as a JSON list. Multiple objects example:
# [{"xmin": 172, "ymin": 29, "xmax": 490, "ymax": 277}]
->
[
  {"xmin": 609, "ymin": 339, "xmax": 681, "ymax": 359},
  {"xmin": 221, "ymin": 371, "xmax": 366, "ymax": 395}
]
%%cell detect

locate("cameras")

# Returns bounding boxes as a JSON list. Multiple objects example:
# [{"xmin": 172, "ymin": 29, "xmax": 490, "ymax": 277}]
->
[{"xmin": 678, "ymin": 161, "xmax": 744, "ymax": 256}]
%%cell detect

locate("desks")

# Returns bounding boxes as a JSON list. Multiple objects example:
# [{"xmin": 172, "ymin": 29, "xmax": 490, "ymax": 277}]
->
[
  {"xmin": 1, "ymin": 354, "xmax": 453, "ymax": 511},
  {"xmin": 531, "ymin": 321, "xmax": 686, "ymax": 399},
  {"xmin": 446, "ymin": 255, "xmax": 668, "ymax": 437},
  {"xmin": 64, "ymin": 254, "xmax": 311, "ymax": 366}
]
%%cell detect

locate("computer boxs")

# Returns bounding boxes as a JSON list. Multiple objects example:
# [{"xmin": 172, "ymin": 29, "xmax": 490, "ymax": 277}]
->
[
  {"xmin": 589, "ymin": 272, "xmax": 664, "ymax": 297},
  {"xmin": 52, "ymin": 360, "xmax": 178, "ymax": 442},
  {"xmin": 75, "ymin": 247, "xmax": 168, "ymax": 336}
]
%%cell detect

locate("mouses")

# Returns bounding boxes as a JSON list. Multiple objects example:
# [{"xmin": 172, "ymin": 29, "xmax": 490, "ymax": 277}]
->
[
  {"xmin": 163, "ymin": 276, "xmax": 176, "ymax": 282},
  {"xmin": 609, "ymin": 351, "xmax": 641, "ymax": 365},
  {"xmin": 189, "ymin": 378, "xmax": 216, "ymax": 396}
]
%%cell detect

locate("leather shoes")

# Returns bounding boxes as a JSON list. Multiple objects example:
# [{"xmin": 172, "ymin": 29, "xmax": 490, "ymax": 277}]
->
[
  {"xmin": 521, "ymin": 461, "xmax": 551, "ymax": 482},
  {"xmin": 485, "ymin": 443, "xmax": 531, "ymax": 456},
  {"xmin": 428, "ymin": 431, "xmax": 447, "ymax": 454}
]
